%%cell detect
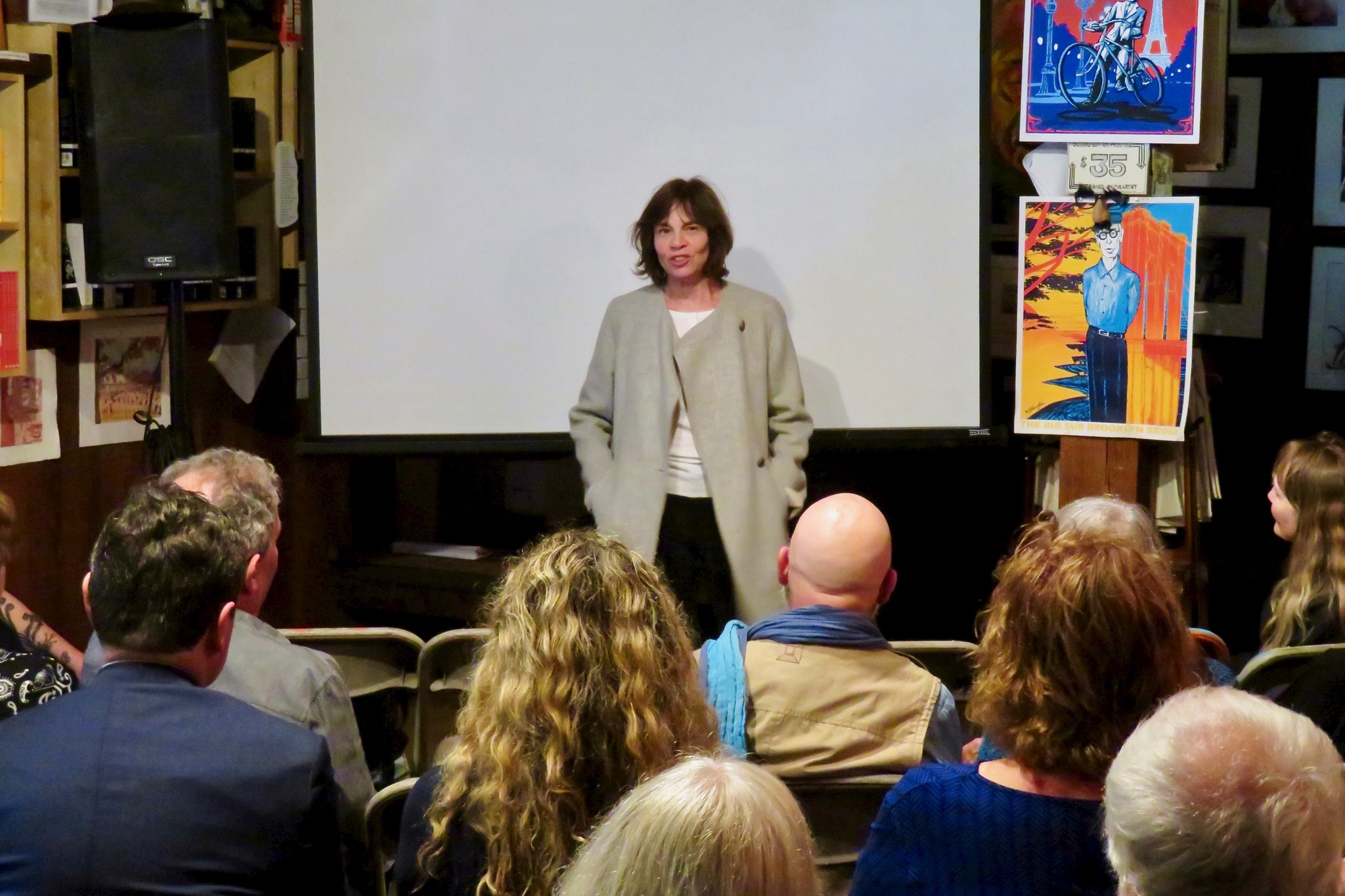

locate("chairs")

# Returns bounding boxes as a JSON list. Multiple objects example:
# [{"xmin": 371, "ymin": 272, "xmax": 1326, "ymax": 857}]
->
[{"xmin": 269, "ymin": 627, "xmax": 1345, "ymax": 896}]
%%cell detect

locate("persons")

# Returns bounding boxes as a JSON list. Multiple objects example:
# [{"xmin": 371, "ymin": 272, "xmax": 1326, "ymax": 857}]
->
[
  {"xmin": 1101, "ymin": 685, "xmax": 1345, "ymax": 896},
  {"xmin": 1261, "ymin": 431, "xmax": 1345, "ymax": 649},
  {"xmin": 689, "ymin": 491, "xmax": 961, "ymax": 896},
  {"xmin": 0, "ymin": 492, "xmax": 86, "ymax": 722},
  {"xmin": 962, "ymin": 493, "xmax": 1235, "ymax": 766},
  {"xmin": 567, "ymin": 176, "xmax": 816, "ymax": 647},
  {"xmin": 0, "ymin": 477, "xmax": 366, "ymax": 896},
  {"xmin": 78, "ymin": 447, "xmax": 377, "ymax": 803},
  {"xmin": 394, "ymin": 525, "xmax": 722, "ymax": 896},
  {"xmin": 843, "ymin": 507, "xmax": 1215, "ymax": 896},
  {"xmin": 548, "ymin": 742, "xmax": 827, "ymax": 896}
]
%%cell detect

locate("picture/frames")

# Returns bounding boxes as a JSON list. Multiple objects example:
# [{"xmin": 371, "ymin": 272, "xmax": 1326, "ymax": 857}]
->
[
  {"xmin": 0, "ymin": 348, "xmax": 61, "ymax": 467},
  {"xmin": 1172, "ymin": 78, "xmax": 1262, "ymax": 187},
  {"xmin": 1312, "ymin": 78, "xmax": 1345, "ymax": 227},
  {"xmin": 1229, "ymin": 0, "xmax": 1345, "ymax": 54},
  {"xmin": 1019, "ymin": 0, "xmax": 1205, "ymax": 144},
  {"xmin": 1305, "ymin": 246, "xmax": 1345, "ymax": 391},
  {"xmin": 79, "ymin": 317, "xmax": 171, "ymax": 448},
  {"xmin": 1014, "ymin": 197, "xmax": 1200, "ymax": 442},
  {"xmin": 1194, "ymin": 205, "xmax": 1270, "ymax": 337}
]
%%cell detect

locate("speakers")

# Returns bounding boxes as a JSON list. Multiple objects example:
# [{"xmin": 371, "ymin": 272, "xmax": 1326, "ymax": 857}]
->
[{"xmin": 70, "ymin": 17, "xmax": 242, "ymax": 286}]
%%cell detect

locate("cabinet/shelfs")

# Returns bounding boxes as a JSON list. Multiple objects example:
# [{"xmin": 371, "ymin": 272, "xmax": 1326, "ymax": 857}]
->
[{"xmin": 0, "ymin": 22, "xmax": 279, "ymax": 377}]
